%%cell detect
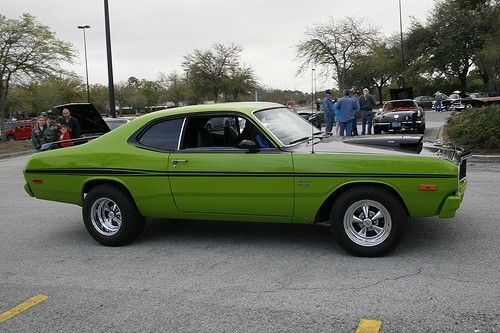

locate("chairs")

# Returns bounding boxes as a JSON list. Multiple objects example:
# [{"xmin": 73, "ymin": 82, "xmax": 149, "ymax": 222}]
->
[
  {"xmin": 224, "ymin": 125, "xmax": 240, "ymax": 145},
  {"xmin": 196, "ymin": 128, "xmax": 215, "ymax": 146}
]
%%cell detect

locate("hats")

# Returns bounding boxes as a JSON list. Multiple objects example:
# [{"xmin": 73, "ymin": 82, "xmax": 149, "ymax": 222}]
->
[
  {"xmin": 325, "ymin": 90, "xmax": 332, "ymax": 94},
  {"xmin": 41, "ymin": 112, "xmax": 46, "ymax": 115}
]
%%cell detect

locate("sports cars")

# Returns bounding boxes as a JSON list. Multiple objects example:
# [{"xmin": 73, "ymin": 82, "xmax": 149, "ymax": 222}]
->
[{"xmin": 22, "ymin": 101, "xmax": 468, "ymax": 258}]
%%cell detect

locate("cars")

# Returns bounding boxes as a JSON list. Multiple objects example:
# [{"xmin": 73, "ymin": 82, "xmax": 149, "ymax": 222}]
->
[
  {"xmin": 298, "ymin": 112, "xmax": 321, "ymax": 130},
  {"xmin": 204, "ymin": 117, "xmax": 247, "ymax": 132},
  {"xmin": 0, "ymin": 119, "xmax": 37, "ymax": 142},
  {"xmin": 52, "ymin": 103, "xmax": 139, "ymax": 144},
  {"xmin": 413, "ymin": 90, "xmax": 497, "ymax": 112},
  {"xmin": 372, "ymin": 86, "xmax": 426, "ymax": 134}
]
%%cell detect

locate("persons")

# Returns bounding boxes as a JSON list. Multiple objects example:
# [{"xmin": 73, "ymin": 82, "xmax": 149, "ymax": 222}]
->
[
  {"xmin": 236, "ymin": 113, "xmax": 275, "ymax": 148},
  {"xmin": 324, "ymin": 89, "xmax": 334, "ymax": 134},
  {"xmin": 382, "ymin": 102, "xmax": 393, "ymax": 112},
  {"xmin": 348, "ymin": 89, "xmax": 360, "ymax": 135},
  {"xmin": 435, "ymin": 91, "xmax": 442, "ymax": 112},
  {"xmin": 32, "ymin": 108, "xmax": 80, "ymax": 152},
  {"xmin": 333, "ymin": 96, "xmax": 340, "ymax": 135},
  {"xmin": 335, "ymin": 89, "xmax": 358, "ymax": 136},
  {"xmin": 288, "ymin": 100, "xmax": 297, "ymax": 113},
  {"xmin": 359, "ymin": 88, "xmax": 375, "ymax": 135},
  {"xmin": 316, "ymin": 100, "xmax": 321, "ymax": 111}
]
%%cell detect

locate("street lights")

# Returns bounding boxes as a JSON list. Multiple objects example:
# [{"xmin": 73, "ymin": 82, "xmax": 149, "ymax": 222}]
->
[{"xmin": 77, "ymin": 25, "xmax": 90, "ymax": 102}]
